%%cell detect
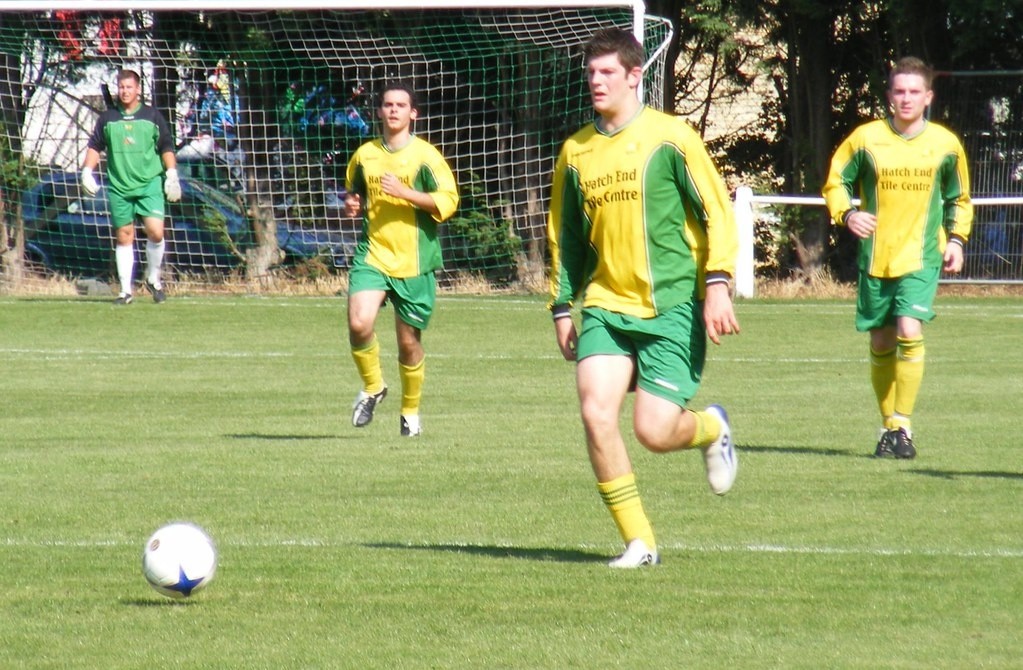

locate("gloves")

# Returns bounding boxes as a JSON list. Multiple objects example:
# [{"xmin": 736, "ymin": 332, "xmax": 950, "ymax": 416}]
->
[
  {"xmin": 81, "ymin": 165, "xmax": 101, "ymax": 198},
  {"xmin": 164, "ymin": 169, "xmax": 182, "ymax": 202}
]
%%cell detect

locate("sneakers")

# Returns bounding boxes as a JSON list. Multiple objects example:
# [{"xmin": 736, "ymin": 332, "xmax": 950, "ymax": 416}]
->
[
  {"xmin": 892, "ymin": 427, "xmax": 916, "ymax": 459},
  {"xmin": 875, "ymin": 427, "xmax": 894, "ymax": 458},
  {"xmin": 113, "ymin": 291, "xmax": 132, "ymax": 305},
  {"xmin": 352, "ymin": 378, "xmax": 388, "ymax": 428},
  {"xmin": 144, "ymin": 278, "xmax": 167, "ymax": 304},
  {"xmin": 400, "ymin": 414, "xmax": 420, "ymax": 437},
  {"xmin": 701, "ymin": 405, "xmax": 737, "ymax": 495},
  {"xmin": 608, "ymin": 539, "xmax": 662, "ymax": 569}
]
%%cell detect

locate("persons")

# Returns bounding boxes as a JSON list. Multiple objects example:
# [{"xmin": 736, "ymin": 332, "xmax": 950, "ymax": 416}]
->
[
  {"xmin": 546, "ymin": 28, "xmax": 742, "ymax": 568},
  {"xmin": 822, "ymin": 56, "xmax": 974, "ymax": 459},
  {"xmin": 344, "ymin": 83, "xmax": 461, "ymax": 437},
  {"xmin": 81, "ymin": 69, "xmax": 182, "ymax": 304}
]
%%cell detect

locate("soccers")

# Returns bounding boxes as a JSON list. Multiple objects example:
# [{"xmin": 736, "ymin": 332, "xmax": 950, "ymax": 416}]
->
[{"xmin": 141, "ymin": 522, "xmax": 219, "ymax": 599}]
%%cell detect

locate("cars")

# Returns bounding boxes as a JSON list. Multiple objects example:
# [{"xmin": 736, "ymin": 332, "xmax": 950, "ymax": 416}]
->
[{"xmin": 7, "ymin": 172, "xmax": 359, "ymax": 283}]
